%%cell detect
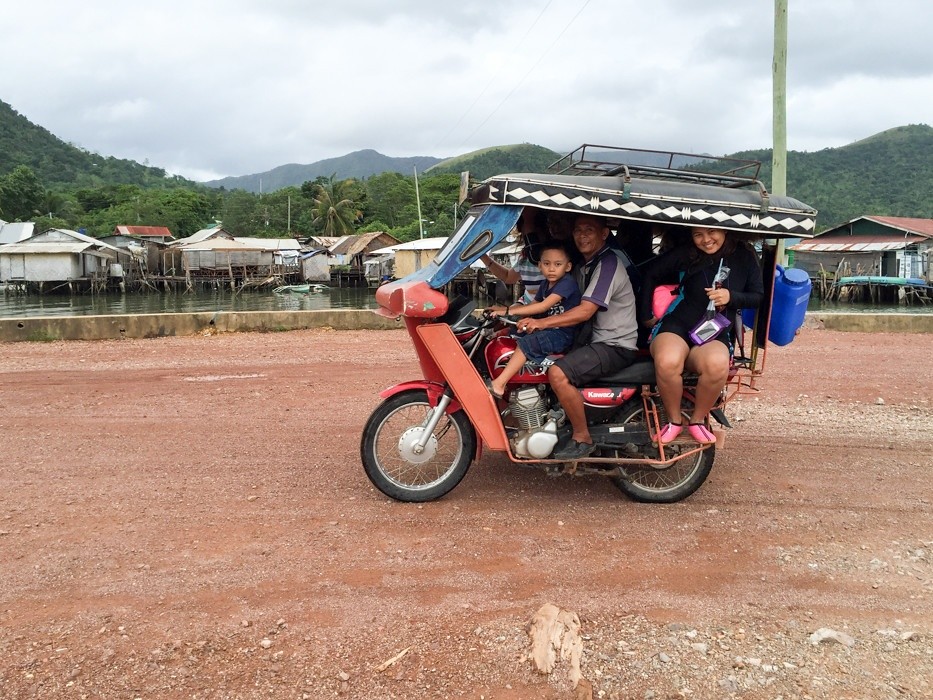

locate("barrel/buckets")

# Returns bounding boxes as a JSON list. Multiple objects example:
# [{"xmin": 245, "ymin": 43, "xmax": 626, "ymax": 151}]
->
[{"xmin": 741, "ymin": 263, "xmax": 811, "ymax": 346}]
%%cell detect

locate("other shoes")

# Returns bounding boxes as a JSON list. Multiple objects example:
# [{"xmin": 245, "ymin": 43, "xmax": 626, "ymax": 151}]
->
[
  {"xmin": 653, "ymin": 422, "xmax": 683, "ymax": 443},
  {"xmin": 688, "ymin": 423, "xmax": 716, "ymax": 444}
]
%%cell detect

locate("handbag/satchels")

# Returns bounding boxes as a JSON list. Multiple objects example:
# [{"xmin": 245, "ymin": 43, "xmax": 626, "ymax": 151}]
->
[
  {"xmin": 571, "ymin": 317, "xmax": 593, "ymax": 349},
  {"xmin": 652, "ymin": 285, "xmax": 680, "ymax": 318}
]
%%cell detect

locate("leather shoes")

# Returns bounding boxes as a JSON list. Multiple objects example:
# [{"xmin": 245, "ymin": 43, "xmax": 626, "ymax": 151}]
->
[{"xmin": 555, "ymin": 439, "xmax": 597, "ymax": 459}]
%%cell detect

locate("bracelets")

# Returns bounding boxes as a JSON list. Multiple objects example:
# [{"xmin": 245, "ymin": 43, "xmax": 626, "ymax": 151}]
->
[{"xmin": 488, "ymin": 260, "xmax": 494, "ymax": 267}]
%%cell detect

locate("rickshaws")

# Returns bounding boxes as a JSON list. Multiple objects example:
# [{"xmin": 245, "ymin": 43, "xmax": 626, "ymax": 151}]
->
[{"xmin": 357, "ymin": 144, "xmax": 820, "ymax": 508}]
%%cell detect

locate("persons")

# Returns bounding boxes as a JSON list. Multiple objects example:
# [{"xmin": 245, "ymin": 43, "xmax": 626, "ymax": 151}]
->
[
  {"xmin": 483, "ymin": 240, "xmax": 581, "ymax": 398},
  {"xmin": 517, "ymin": 214, "xmax": 638, "ymax": 460},
  {"xmin": 630, "ymin": 227, "xmax": 764, "ymax": 444},
  {"xmin": 480, "ymin": 206, "xmax": 680, "ymax": 321}
]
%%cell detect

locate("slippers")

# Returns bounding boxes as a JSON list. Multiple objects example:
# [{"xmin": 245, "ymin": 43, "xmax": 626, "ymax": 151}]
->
[{"xmin": 487, "ymin": 378, "xmax": 503, "ymax": 398}]
